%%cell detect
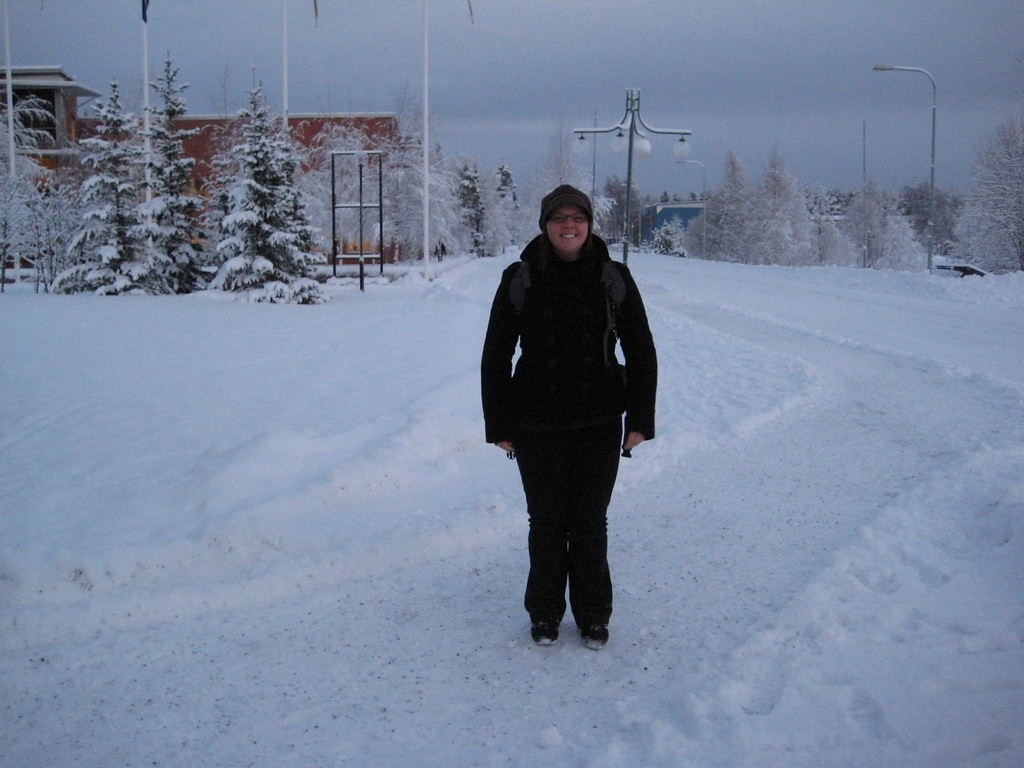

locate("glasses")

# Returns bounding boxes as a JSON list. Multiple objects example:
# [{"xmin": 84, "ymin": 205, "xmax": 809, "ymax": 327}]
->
[{"xmin": 548, "ymin": 208, "xmax": 589, "ymax": 224}]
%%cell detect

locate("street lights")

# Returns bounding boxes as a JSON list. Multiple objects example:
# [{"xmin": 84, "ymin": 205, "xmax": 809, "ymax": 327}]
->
[
  {"xmin": 621, "ymin": 183, "xmax": 643, "ymax": 253},
  {"xmin": 574, "ymin": 86, "xmax": 693, "ymax": 267},
  {"xmin": 871, "ymin": 65, "xmax": 937, "ymax": 273},
  {"xmin": 676, "ymin": 159, "xmax": 708, "ymax": 260}
]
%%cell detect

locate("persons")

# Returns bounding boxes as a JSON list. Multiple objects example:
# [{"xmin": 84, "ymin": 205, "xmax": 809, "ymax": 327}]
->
[{"xmin": 481, "ymin": 186, "xmax": 659, "ymax": 650}]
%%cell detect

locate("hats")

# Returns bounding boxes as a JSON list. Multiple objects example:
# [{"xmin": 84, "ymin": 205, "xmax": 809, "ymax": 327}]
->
[{"xmin": 539, "ymin": 185, "xmax": 593, "ymax": 231}]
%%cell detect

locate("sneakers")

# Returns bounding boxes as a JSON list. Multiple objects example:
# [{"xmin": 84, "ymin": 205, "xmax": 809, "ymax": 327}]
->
[
  {"xmin": 580, "ymin": 621, "xmax": 609, "ymax": 649},
  {"xmin": 530, "ymin": 612, "xmax": 560, "ymax": 645}
]
